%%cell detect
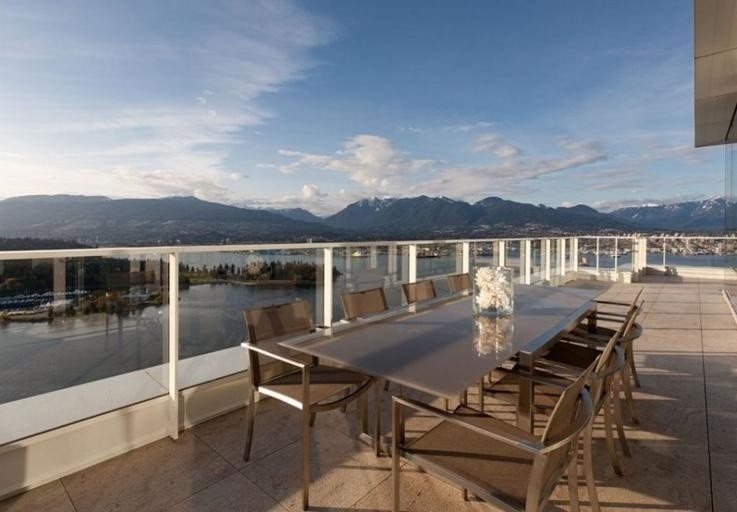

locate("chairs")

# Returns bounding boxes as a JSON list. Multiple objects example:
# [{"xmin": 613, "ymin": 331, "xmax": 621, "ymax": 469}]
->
[
  {"xmin": 337, "ymin": 286, "xmax": 450, "ymax": 439},
  {"xmin": 391, "ymin": 356, "xmax": 602, "ymax": 510},
  {"xmin": 242, "ymin": 299, "xmax": 381, "ymax": 510},
  {"xmin": 477, "ymin": 320, "xmax": 631, "ymax": 478},
  {"xmin": 506, "ymin": 300, "xmax": 647, "ymax": 427},
  {"xmin": 401, "ymin": 279, "xmax": 438, "ymax": 304},
  {"xmin": 564, "ymin": 286, "xmax": 647, "ymax": 390},
  {"xmin": 446, "ymin": 272, "xmax": 471, "ymax": 291}
]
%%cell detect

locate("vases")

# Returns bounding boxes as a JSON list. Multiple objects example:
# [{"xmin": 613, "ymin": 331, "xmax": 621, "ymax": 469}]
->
[{"xmin": 473, "ymin": 264, "xmax": 514, "ymax": 317}]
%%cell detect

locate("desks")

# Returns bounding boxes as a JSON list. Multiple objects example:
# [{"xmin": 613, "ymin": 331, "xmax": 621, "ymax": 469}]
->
[{"xmin": 276, "ymin": 281, "xmax": 606, "ymax": 512}]
